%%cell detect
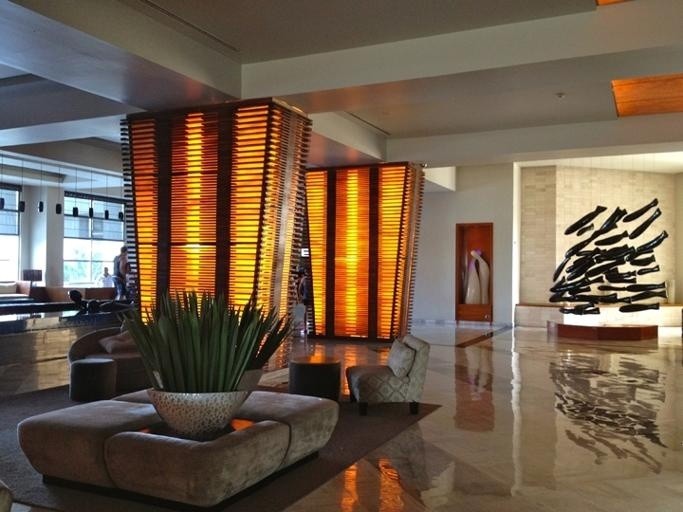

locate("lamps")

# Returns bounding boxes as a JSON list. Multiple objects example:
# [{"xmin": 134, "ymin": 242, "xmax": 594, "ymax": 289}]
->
[
  {"xmin": 19, "ymin": 160, "xmax": 25, "ymax": 212},
  {"xmin": 0, "ymin": 154, "xmax": 4, "ymax": 209},
  {"xmin": 73, "ymin": 166, "xmax": 78, "ymax": 216},
  {"xmin": 56, "ymin": 161, "xmax": 62, "ymax": 214},
  {"xmin": 104, "ymin": 172, "xmax": 109, "ymax": 219},
  {"xmin": 38, "ymin": 158, "xmax": 43, "ymax": 213},
  {"xmin": 118, "ymin": 177, "xmax": 123, "ymax": 220},
  {"xmin": 89, "ymin": 168, "xmax": 93, "ymax": 218}
]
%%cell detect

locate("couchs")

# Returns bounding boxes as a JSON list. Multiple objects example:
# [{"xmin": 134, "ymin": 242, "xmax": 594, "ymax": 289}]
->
[{"xmin": 68, "ymin": 327, "xmax": 153, "ymax": 396}]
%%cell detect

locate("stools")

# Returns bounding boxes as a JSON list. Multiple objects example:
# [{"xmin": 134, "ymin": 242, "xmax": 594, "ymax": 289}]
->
[
  {"xmin": 287, "ymin": 355, "xmax": 342, "ymax": 400},
  {"xmin": 69, "ymin": 358, "xmax": 118, "ymax": 404}
]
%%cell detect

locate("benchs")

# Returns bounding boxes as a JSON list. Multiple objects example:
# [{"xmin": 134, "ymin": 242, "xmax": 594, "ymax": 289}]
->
[{"xmin": 17, "ymin": 384, "xmax": 341, "ymax": 511}]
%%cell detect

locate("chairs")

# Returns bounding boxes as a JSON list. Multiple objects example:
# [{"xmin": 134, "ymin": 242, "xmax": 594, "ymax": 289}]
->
[{"xmin": 345, "ymin": 336, "xmax": 431, "ymax": 416}]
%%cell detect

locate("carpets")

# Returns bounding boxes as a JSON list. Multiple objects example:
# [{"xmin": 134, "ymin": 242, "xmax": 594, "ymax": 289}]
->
[{"xmin": 0, "ymin": 385, "xmax": 442, "ymax": 512}]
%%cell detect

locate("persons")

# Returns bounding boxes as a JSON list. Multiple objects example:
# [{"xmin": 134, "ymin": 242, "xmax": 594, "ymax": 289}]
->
[
  {"xmin": 299, "ymin": 268, "xmax": 312, "ymax": 334},
  {"xmin": 113, "ymin": 247, "xmax": 128, "ymax": 301},
  {"xmin": 96, "ymin": 267, "xmax": 113, "ymax": 287}
]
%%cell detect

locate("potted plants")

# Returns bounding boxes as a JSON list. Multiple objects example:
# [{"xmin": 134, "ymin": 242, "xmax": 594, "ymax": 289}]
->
[
  {"xmin": 235, "ymin": 304, "xmax": 299, "ymax": 410},
  {"xmin": 115, "ymin": 288, "xmax": 261, "ymax": 437}
]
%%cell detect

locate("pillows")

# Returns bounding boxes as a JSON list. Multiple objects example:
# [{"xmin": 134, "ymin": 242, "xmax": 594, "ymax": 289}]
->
[
  {"xmin": 0, "ymin": 282, "xmax": 18, "ymax": 294},
  {"xmin": 97, "ymin": 330, "xmax": 139, "ymax": 354},
  {"xmin": 387, "ymin": 339, "xmax": 417, "ymax": 378},
  {"xmin": 120, "ymin": 319, "xmax": 135, "ymax": 333}
]
